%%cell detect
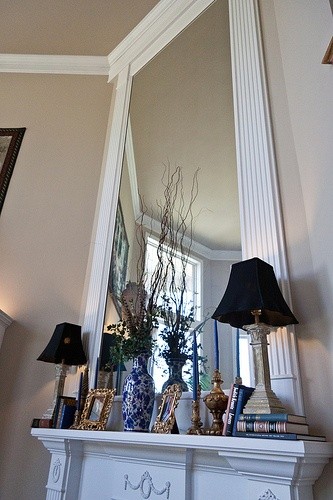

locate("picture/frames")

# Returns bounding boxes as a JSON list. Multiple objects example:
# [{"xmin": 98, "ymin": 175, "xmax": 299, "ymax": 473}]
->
[
  {"xmin": 156, "ymin": 384, "xmax": 182, "ymax": 434},
  {"xmin": 108, "ymin": 200, "xmax": 130, "ymax": 322},
  {"xmin": 77, "ymin": 388, "xmax": 117, "ymax": 431},
  {"xmin": 0, "ymin": 127, "xmax": 27, "ymax": 214}
]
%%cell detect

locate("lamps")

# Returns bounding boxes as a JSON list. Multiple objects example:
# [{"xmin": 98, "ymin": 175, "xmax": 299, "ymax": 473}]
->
[
  {"xmin": 211, "ymin": 257, "xmax": 299, "ymax": 415},
  {"xmin": 36, "ymin": 322, "xmax": 88, "ymax": 428}
]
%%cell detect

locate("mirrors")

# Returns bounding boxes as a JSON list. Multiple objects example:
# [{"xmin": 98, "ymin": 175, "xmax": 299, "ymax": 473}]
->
[{"xmin": 80, "ymin": 0, "xmax": 304, "ymax": 418}]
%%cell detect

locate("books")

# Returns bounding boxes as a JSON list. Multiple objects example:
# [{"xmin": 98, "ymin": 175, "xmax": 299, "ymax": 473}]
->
[
  {"xmin": 222, "ymin": 383, "xmax": 327, "ymax": 442},
  {"xmin": 31, "ymin": 395, "xmax": 76, "ymax": 429}
]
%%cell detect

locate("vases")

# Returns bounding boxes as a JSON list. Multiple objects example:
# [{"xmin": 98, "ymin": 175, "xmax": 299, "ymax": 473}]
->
[
  {"xmin": 121, "ymin": 353, "xmax": 156, "ymax": 431},
  {"xmin": 161, "ymin": 357, "xmax": 188, "ymax": 391}
]
